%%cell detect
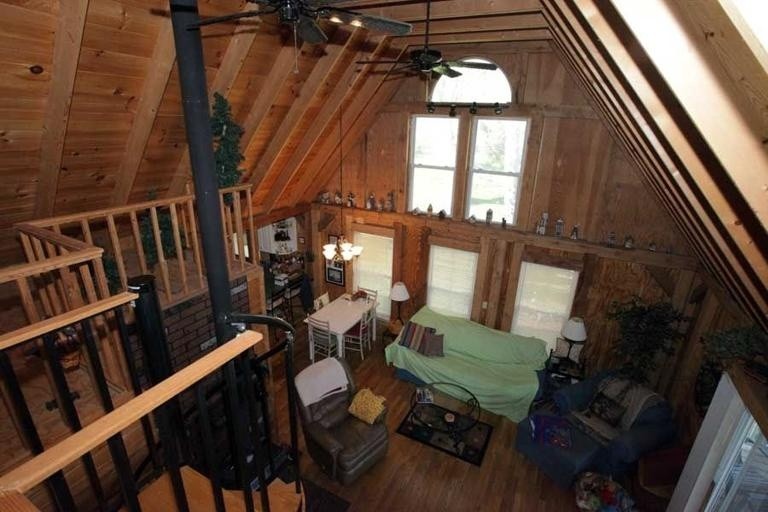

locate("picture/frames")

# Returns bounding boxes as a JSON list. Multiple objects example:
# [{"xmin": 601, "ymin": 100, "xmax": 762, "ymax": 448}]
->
[{"xmin": 325, "ymin": 256, "xmax": 345, "ymax": 287}]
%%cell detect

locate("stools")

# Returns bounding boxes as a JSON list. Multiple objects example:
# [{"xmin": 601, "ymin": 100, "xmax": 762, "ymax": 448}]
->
[{"xmin": 515, "ymin": 407, "xmax": 599, "ymax": 492}]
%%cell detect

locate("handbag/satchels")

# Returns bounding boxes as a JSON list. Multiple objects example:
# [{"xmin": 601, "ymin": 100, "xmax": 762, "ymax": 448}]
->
[{"xmin": 589, "ymin": 376, "xmax": 633, "ymax": 428}]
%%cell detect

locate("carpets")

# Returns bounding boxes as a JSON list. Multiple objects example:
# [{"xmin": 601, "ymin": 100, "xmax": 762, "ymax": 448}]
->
[{"xmin": 396, "ymin": 402, "xmax": 493, "ymax": 466}]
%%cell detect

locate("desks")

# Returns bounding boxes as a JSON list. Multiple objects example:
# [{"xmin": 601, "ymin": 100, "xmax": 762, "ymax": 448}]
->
[
  {"xmin": 542, "ymin": 350, "xmax": 588, "ymax": 397},
  {"xmin": 382, "ymin": 320, "xmax": 404, "ymax": 345}
]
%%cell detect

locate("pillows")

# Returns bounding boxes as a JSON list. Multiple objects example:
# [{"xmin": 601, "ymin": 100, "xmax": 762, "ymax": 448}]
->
[
  {"xmin": 398, "ymin": 321, "xmax": 436, "ymax": 354},
  {"xmin": 423, "ymin": 333, "xmax": 444, "ymax": 356},
  {"xmin": 589, "ymin": 391, "xmax": 627, "ymax": 427}
]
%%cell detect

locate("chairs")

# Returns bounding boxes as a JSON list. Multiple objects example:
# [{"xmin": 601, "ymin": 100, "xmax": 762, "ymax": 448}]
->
[
  {"xmin": 293, "ymin": 356, "xmax": 389, "ymax": 487},
  {"xmin": 265, "ymin": 268, "xmax": 380, "ymax": 364},
  {"xmin": 553, "ymin": 366, "xmax": 674, "ymax": 480}
]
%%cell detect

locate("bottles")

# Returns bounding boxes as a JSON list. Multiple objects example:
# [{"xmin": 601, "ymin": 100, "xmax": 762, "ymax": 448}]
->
[
  {"xmin": 366, "ymin": 294, "xmax": 370, "ymax": 306},
  {"xmin": 555, "ymin": 220, "xmax": 564, "ymax": 237}
]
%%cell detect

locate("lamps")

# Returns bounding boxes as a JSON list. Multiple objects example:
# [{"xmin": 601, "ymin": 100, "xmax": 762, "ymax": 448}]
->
[
  {"xmin": 561, "ymin": 317, "xmax": 587, "ymax": 368},
  {"xmin": 322, "ymin": 105, "xmax": 363, "ymax": 262},
  {"xmin": 427, "ymin": 103, "xmax": 509, "ymax": 117},
  {"xmin": 390, "ymin": 281, "xmax": 410, "ymax": 326}
]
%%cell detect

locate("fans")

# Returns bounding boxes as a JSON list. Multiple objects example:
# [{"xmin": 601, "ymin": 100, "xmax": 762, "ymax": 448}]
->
[
  {"xmin": 355, "ymin": 1, "xmax": 498, "ymax": 78},
  {"xmin": 187, "ymin": 0, "xmax": 413, "ymax": 44}
]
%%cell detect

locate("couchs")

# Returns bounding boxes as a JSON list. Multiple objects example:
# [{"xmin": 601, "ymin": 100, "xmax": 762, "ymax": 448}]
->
[{"xmin": 385, "ymin": 304, "xmax": 547, "ymax": 423}]
